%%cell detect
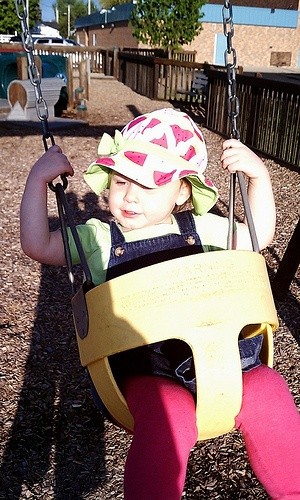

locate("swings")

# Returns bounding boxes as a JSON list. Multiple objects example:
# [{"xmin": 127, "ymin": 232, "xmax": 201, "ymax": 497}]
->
[{"xmin": 15, "ymin": 0, "xmax": 281, "ymax": 440}]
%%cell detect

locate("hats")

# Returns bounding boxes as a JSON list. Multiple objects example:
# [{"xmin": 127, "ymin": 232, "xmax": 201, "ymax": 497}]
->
[{"xmin": 82, "ymin": 108, "xmax": 219, "ymax": 216}]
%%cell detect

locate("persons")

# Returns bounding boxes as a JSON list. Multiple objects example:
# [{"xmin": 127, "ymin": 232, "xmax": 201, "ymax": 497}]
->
[{"xmin": 20, "ymin": 108, "xmax": 300, "ymax": 500}]
